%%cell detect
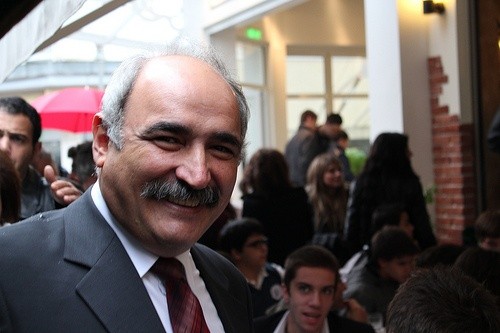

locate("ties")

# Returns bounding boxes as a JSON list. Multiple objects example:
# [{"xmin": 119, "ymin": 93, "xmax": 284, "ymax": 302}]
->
[{"xmin": 148, "ymin": 257, "xmax": 210, "ymax": 333}]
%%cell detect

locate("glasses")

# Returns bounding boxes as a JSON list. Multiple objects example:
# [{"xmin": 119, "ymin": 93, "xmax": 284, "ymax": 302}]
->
[{"xmin": 244, "ymin": 239, "xmax": 271, "ymax": 248}]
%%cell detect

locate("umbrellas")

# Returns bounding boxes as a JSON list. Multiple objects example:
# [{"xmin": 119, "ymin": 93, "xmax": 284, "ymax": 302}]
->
[{"xmin": 31, "ymin": 87, "xmax": 105, "ymax": 134}]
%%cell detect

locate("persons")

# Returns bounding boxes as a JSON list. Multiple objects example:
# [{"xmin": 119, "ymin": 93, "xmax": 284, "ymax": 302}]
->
[
  {"xmin": 0, "ymin": 39, "xmax": 251, "ymax": 333},
  {"xmin": 0, "ymin": 96, "xmax": 98, "ymax": 228},
  {"xmin": 199, "ymin": 110, "xmax": 500, "ymax": 333}
]
